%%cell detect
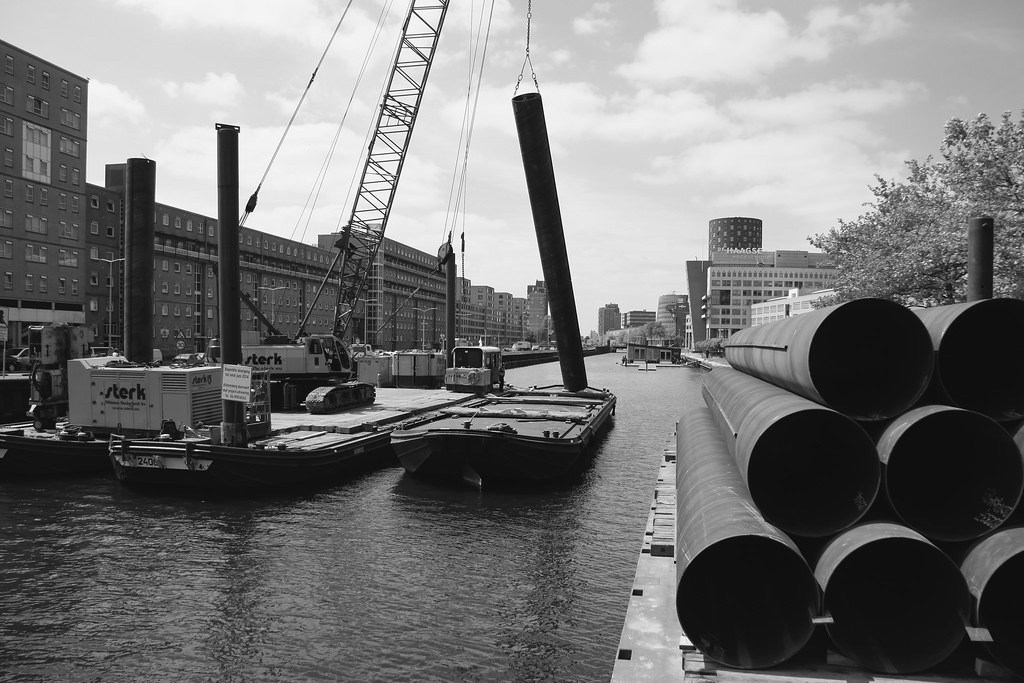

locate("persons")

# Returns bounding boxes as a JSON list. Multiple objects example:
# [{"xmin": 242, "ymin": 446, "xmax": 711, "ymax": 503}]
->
[
  {"xmin": 705, "ymin": 348, "xmax": 709, "ymax": 358},
  {"xmin": 499, "ymin": 362, "xmax": 506, "ymax": 392}
]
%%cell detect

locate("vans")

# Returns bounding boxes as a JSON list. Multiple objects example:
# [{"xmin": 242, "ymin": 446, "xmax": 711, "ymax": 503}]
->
[
  {"xmin": 90, "ymin": 346, "xmax": 114, "ymax": 358},
  {"xmin": 153, "ymin": 349, "xmax": 163, "ymax": 366}
]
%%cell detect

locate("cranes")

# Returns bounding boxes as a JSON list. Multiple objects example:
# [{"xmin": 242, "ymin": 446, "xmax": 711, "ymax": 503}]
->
[{"xmin": 205, "ymin": 0, "xmax": 542, "ymax": 416}]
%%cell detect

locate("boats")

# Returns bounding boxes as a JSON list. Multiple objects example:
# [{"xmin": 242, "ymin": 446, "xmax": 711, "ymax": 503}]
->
[
  {"xmin": 0, "ymin": 343, "xmax": 514, "ymax": 497},
  {"xmin": 390, "ymin": 380, "xmax": 618, "ymax": 494}
]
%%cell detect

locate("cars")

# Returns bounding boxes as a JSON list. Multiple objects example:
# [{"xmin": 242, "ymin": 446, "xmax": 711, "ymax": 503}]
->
[
  {"xmin": 7, "ymin": 347, "xmax": 37, "ymax": 370},
  {"xmin": 0, "ymin": 352, "xmax": 18, "ymax": 372},
  {"xmin": 170, "ymin": 354, "xmax": 205, "ymax": 367},
  {"xmin": 349, "ymin": 340, "xmax": 558, "ymax": 361}
]
